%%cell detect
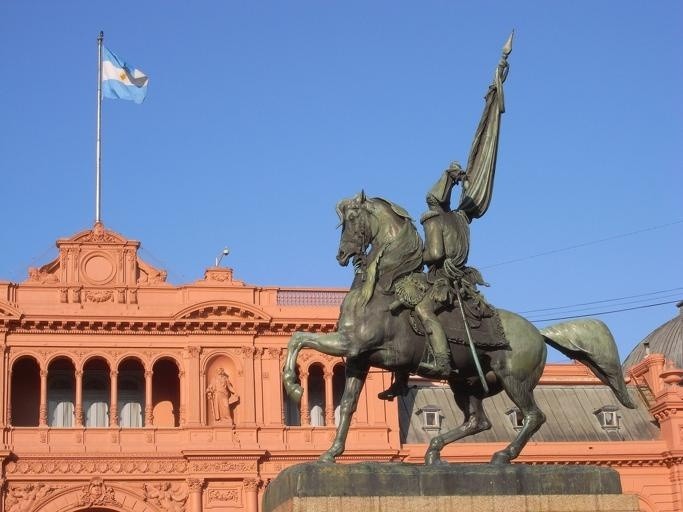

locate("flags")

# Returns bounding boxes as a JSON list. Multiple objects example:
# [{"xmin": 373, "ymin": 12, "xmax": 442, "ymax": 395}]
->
[
  {"xmin": 456, "ymin": 73, "xmax": 517, "ymax": 216},
  {"xmin": 100, "ymin": 43, "xmax": 148, "ymax": 105}
]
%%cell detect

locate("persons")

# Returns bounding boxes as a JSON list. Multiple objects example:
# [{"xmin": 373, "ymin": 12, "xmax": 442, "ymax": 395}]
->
[
  {"xmin": 207, "ymin": 367, "xmax": 239, "ymax": 427},
  {"xmin": 376, "ymin": 158, "xmax": 491, "ymax": 400}
]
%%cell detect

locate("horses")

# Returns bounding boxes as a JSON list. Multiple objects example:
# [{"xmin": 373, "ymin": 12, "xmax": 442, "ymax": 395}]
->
[{"xmin": 281, "ymin": 188, "xmax": 638, "ymax": 465}]
